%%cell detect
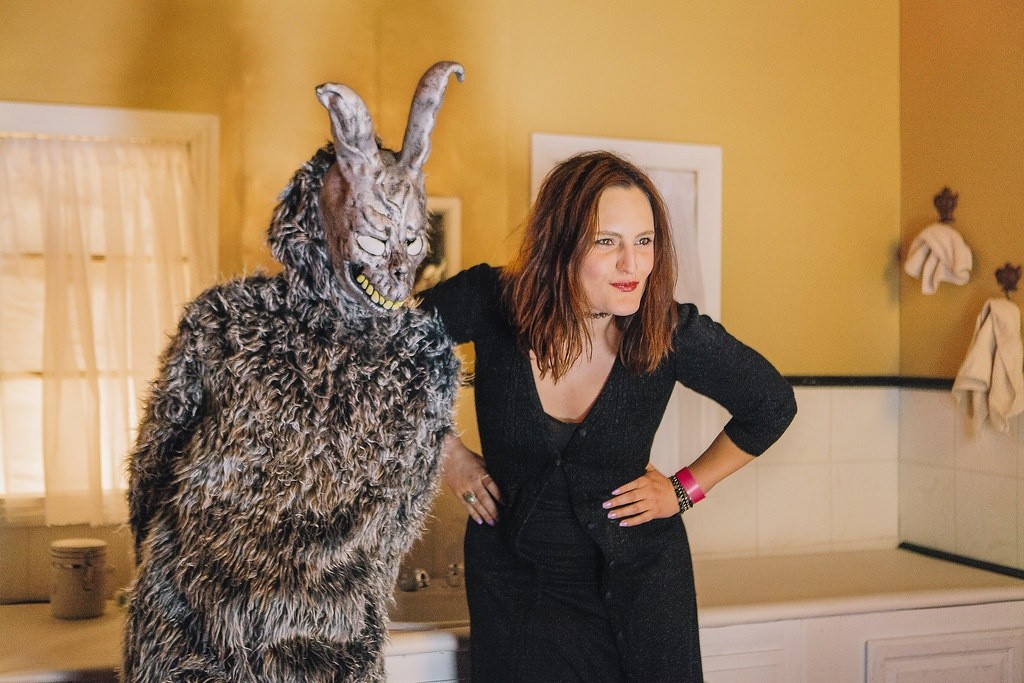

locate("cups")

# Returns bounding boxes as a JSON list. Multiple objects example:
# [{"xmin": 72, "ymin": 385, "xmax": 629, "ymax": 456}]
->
[{"xmin": 48, "ymin": 539, "xmax": 108, "ymax": 619}]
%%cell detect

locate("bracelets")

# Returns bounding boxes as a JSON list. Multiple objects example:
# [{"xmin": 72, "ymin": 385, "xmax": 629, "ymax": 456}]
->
[
  {"xmin": 676, "ymin": 466, "xmax": 705, "ymax": 504},
  {"xmin": 669, "ymin": 473, "xmax": 693, "ymax": 514}
]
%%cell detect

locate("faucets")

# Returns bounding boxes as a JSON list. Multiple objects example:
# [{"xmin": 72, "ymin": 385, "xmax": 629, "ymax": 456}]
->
[{"xmin": 399, "ymin": 567, "xmax": 430, "ymax": 592}]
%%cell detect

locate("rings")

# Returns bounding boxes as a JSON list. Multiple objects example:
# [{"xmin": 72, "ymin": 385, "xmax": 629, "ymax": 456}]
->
[
  {"xmin": 481, "ymin": 473, "xmax": 488, "ymax": 479},
  {"xmin": 462, "ymin": 492, "xmax": 476, "ymax": 503}
]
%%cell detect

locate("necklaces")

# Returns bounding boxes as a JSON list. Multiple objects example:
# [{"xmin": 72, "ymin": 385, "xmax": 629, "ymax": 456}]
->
[{"xmin": 582, "ymin": 310, "xmax": 613, "ymax": 319}]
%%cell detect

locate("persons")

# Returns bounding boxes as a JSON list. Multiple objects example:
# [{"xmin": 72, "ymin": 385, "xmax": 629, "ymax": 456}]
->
[{"xmin": 414, "ymin": 148, "xmax": 797, "ymax": 683}]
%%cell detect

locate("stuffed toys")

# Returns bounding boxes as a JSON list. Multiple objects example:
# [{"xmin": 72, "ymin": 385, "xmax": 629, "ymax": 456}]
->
[{"xmin": 121, "ymin": 59, "xmax": 464, "ymax": 683}]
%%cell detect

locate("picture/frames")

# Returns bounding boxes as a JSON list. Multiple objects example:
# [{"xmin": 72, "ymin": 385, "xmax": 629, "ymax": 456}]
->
[
  {"xmin": 531, "ymin": 134, "xmax": 722, "ymax": 324},
  {"xmin": 417, "ymin": 196, "xmax": 461, "ymax": 295}
]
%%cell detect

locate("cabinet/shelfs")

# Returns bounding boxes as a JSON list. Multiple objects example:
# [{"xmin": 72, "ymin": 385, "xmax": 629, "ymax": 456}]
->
[{"xmin": 383, "ymin": 598, "xmax": 1023, "ymax": 682}]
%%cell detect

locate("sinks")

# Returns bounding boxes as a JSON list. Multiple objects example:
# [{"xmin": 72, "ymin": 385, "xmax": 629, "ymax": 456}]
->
[{"xmin": 388, "ymin": 593, "xmax": 469, "ymax": 631}]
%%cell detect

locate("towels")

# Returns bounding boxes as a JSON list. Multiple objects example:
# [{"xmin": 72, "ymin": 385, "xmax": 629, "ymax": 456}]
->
[
  {"xmin": 949, "ymin": 297, "xmax": 1024, "ymax": 442},
  {"xmin": 903, "ymin": 224, "xmax": 973, "ymax": 296}
]
